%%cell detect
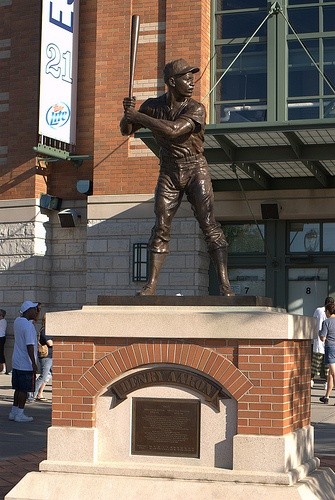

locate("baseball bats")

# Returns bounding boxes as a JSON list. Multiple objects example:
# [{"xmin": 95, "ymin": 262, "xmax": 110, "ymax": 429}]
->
[{"xmin": 129, "ymin": 14, "xmax": 140, "ymax": 96}]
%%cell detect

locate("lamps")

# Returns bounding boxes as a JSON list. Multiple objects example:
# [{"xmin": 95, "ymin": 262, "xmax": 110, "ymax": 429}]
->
[
  {"xmin": 57, "ymin": 209, "xmax": 77, "ymax": 229},
  {"xmin": 261, "ymin": 203, "xmax": 280, "ymax": 219},
  {"xmin": 132, "ymin": 243, "xmax": 149, "ymax": 282},
  {"xmin": 40, "ymin": 193, "xmax": 63, "ymax": 211}
]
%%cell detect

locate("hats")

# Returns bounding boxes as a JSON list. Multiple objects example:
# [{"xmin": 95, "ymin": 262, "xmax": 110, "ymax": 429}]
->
[{"xmin": 20, "ymin": 301, "xmax": 39, "ymax": 313}]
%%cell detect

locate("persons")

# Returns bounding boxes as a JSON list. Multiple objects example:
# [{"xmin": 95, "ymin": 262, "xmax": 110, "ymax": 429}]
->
[
  {"xmin": 7, "ymin": 301, "xmax": 38, "ymax": 422},
  {"xmin": 27, "ymin": 302, "xmax": 53, "ymax": 404},
  {"xmin": 0, "ymin": 309, "xmax": 7, "ymax": 375},
  {"xmin": 310, "ymin": 297, "xmax": 334, "ymax": 390},
  {"xmin": 120, "ymin": 58, "xmax": 234, "ymax": 297},
  {"xmin": 318, "ymin": 304, "xmax": 335, "ymax": 403}
]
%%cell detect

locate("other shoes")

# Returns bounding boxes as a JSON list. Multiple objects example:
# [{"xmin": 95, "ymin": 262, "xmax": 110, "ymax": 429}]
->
[
  {"xmin": 25, "ymin": 400, "xmax": 38, "ymax": 404},
  {"xmin": 324, "ymin": 382, "xmax": 327, "ymax": 389},
  {"xmin": 310, "ymin": 380, "xmax": 314, "ymax": 388},
  {"xmin": 319, "ymin": 396, "xmax": 329, "ymax": 404},
  {"xmin": 0, "ymin": 370, "xmax": 8, "ymax": 375}
]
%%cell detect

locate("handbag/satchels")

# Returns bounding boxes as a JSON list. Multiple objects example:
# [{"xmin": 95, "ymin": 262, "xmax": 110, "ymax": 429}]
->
[{"xmin": 38, "ymin": 342, "xmax": 48, "ymax": 357}]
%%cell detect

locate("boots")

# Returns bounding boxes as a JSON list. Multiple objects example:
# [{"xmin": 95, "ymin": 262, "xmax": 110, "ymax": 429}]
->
[
  {"xmin": 8, "ymin": 406, "xmax": 18, "ymax": 419},
  {"xmin": 14, "ymin": 407, "xmax": 33, "ymax": 422}
]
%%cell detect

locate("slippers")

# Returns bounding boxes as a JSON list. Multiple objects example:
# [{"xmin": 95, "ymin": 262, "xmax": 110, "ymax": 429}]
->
[{"xmin": 36, "ymin": 397, "xmax": 47, "ymax": 401}]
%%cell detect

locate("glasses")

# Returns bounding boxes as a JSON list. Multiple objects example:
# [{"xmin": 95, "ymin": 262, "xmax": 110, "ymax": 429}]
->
[{"xmin": 30, "ymin": 307, "xmax": 37, "ymax": 312}]
